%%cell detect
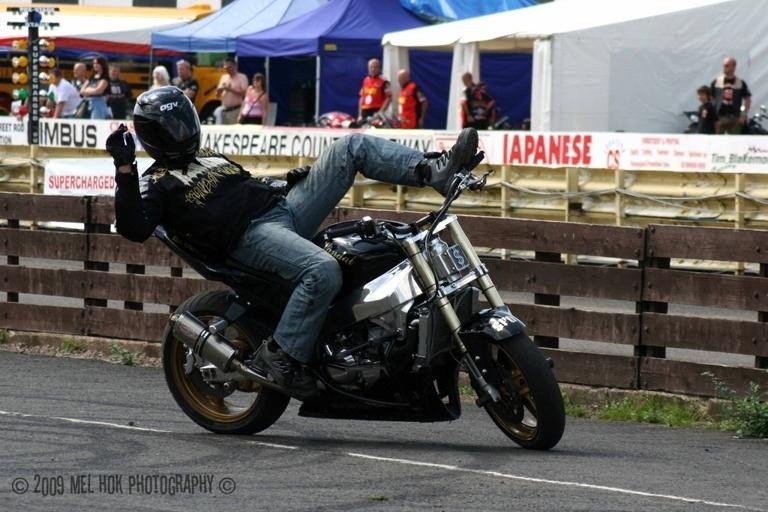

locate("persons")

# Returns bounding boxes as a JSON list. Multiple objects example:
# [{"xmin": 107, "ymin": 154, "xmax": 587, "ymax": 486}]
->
[
  {"xmin": 460, "ymin": 71, "xmax": 495, "ymax": 130},
  {"xmin": 359, "ymin": 58, "xmax": 391, "ymax": 122},
  {"xmin": 710, "ymin": 57, "xmax": 751, "ymax": 134},
  {"xmin": 397, "ymin": 69, "xmax": 428, "ymax": 129},
  {"xmin": 11, "ymin": 55, "xmax": 268, "ymax": 127},
  {"xmin": 696, "ymin": 85, "xmax": 721, "ymax": 134},
  {"xmin": 104, "ymin": 83, "xmax": 479, "ymax": 400}
]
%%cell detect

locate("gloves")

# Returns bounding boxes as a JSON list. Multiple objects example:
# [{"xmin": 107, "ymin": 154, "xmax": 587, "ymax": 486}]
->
[
  {"xmin": 106, "ymin": 123, "xmax": 136, "ymax": 169},
  {"xmin": 286, "ymin": 164, "xmax": 311, "ymax": 192}
]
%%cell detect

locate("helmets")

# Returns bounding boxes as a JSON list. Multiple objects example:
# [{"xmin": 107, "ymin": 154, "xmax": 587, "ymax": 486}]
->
[{"xmin": 132, "ymin": 84, "xmax": 203, "ymax": 166}]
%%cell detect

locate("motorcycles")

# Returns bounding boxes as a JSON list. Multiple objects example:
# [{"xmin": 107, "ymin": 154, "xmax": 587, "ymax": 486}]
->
[
  {"xmin": 316, "ymin": 104, "xmax": 397, "ymax": 128},
  {"xmin": 681, "ymin": 103, "xmax": 767, "ymax": 134},
  {"xmin": 148, "ymin": 144, "xmax": 567, "ymax": 451}
]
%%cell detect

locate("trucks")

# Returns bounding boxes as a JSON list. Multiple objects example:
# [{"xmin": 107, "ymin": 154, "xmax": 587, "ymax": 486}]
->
[{"xmin": 0, "ymin": 45, "xmax": 233, "ymax": 126}]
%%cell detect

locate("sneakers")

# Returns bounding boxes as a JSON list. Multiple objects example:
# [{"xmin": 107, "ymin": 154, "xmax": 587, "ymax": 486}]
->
[{"xmin": 247, "ymin": 335, "xmax": 316, "ymax": 391}]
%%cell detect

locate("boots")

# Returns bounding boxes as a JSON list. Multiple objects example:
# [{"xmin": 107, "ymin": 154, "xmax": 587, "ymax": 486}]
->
[{"xmin": 416, "ymin": 125, "xmax": 479, "ymax": 201}]
